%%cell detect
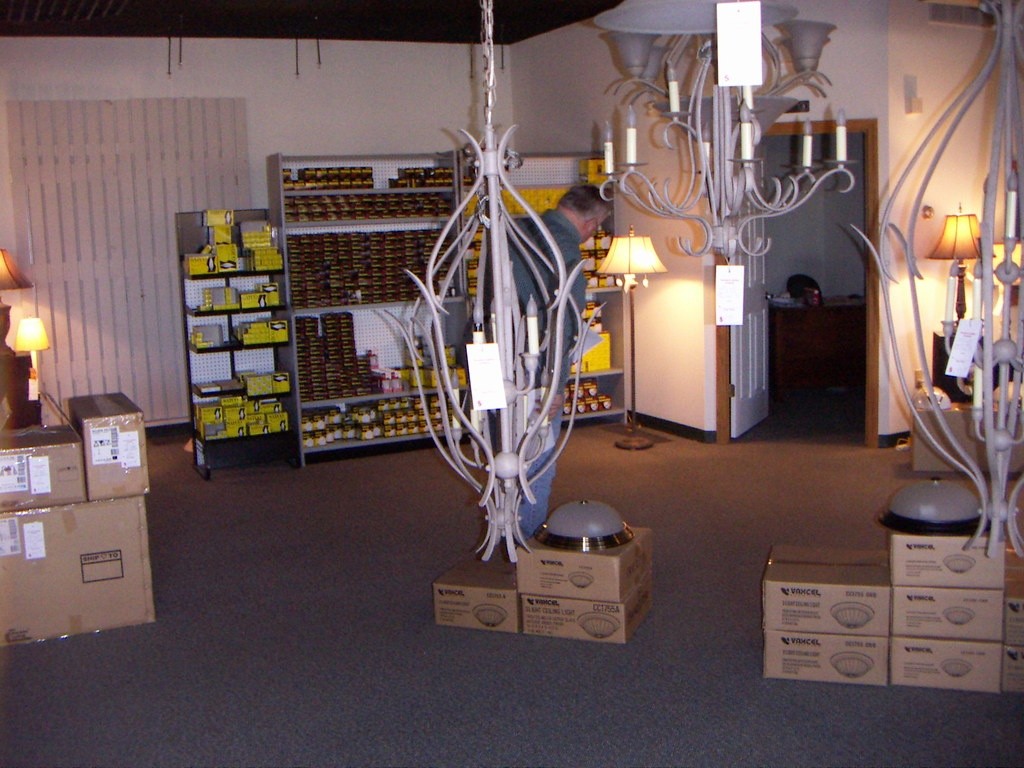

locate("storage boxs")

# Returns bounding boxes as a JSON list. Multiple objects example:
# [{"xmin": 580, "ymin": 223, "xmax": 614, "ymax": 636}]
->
[
  {"xmin": 181, "ymin": 209, "xmax": 291, "ymax": 441},
  {"xmin": 760, "ymin": 523, "xmax": 1024, "ymax": 695},
  {"xmin": 466, "ymin": 158, "xmax": 611, "ymax": 416},
  {"xmin": 0, "ymin": 422, "xmax": 87, "ymax": 513},
  {"xmin": 299, "ymin": 347, "xmax": 468, "ymax": 447},
  {"xmin": 0, "ymin": 495, "xmax": 156, "ymax": 648},
  {"xmin": 430, "ymin": 552, "xmax": 524, "ymax": 634},
  {"xmin": 519, "ymin": 570, "xmax": 656, "ymax": 644},
  {"xmin": 67, "ymin": 393, "xmax": 150, "ymax": 502},
  {"xmin": 514, "ymin": 527, "xmax": 653, "ymax": 601}
]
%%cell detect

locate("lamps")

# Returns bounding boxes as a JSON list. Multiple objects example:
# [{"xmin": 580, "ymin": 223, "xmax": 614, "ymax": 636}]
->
[
  {"xmin": 594, "ymin": 222, "xmax": 668, "ymax": 452},
  {"xmin": 924, "ymin": 202, "xmax": 996, "ymax": 338},
  {"xmin": 0, "ymin": 248, "xmax": 33, "ymax": 351},
  {"xmin": 593, "ymin": 0, "xmax": 855, "ymax": 265},
  {"xmin": 15, "ymin": 317, "xmax": 49, "ymax": 372}
]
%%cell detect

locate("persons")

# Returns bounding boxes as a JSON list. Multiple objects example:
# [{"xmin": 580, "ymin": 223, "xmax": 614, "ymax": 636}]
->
[{"xmin": 477, "ymin": 183, "xmax": 613, "ymax": 547}]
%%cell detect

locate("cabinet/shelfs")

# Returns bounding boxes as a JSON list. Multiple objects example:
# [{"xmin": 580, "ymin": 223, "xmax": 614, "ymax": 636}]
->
[
  {"xmin": 266, "ymin": 148, "xmax": 466, "ymax": 467},
  {"xmin": 768, "ymin": 301, "xmax": 866, "ymax": 397},
  {"xmin": 459, "ymin": 149, "xmax": 629, "ymax": 435},
  {"xmin": 175, "ymin": 208, "xmax": 301, "ymax": 482}
]
